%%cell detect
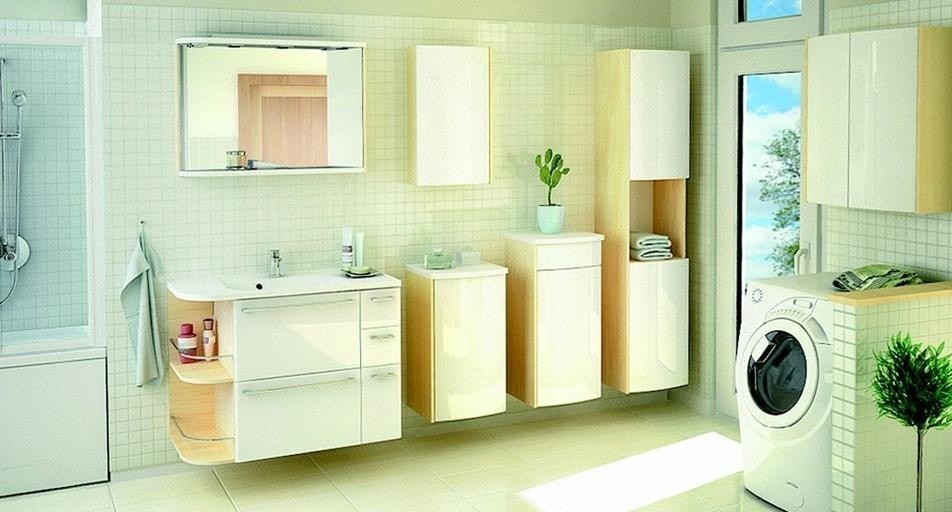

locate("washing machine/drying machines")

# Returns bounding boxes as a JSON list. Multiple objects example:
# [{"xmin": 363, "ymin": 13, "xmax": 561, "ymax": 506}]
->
[{"xmin": 734, "ymin": 270, "xmax": 846, "ymax": 512}]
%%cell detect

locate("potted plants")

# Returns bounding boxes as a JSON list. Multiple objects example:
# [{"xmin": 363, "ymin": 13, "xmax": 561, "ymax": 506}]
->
[{"xmin": 535, "ymin": 149, "xmax": 570, "ymax": 235}]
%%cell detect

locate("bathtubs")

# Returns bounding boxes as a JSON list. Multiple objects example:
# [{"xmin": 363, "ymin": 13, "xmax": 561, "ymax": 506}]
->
[{"xmin": 2, "ymin": 334, "xmax": 107, "ymax": 368}]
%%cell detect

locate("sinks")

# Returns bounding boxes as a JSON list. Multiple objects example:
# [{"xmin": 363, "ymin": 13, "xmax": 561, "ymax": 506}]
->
[{"xmin": 218, "ymin": 273, "xmax": 355, "ymax": 293}]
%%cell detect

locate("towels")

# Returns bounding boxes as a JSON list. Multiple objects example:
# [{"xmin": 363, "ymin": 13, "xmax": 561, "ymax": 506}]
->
[
  {"xmin": 830, "ymin": 265, "xmax": 923, "ymax": 293},
  {"xmin": 119, "ymin": 220, "xmax": 165, "ymax": 387},
  {"xmin": 631, "ymin": 232, "xmax": 673, "ymax": 260}
]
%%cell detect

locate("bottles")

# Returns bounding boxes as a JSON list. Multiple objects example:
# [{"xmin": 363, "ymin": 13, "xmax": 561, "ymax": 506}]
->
[{"xmin": 226, "ymin": 151, "xmax": 246, "ymax": 169}]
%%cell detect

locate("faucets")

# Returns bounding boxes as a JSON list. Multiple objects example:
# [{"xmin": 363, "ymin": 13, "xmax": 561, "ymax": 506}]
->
[{"xmin": 266, "ymin": 249, "xmax": 283, "ymax": 278}]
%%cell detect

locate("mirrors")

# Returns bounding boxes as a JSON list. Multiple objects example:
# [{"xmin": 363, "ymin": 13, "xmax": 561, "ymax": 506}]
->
[{"xmin": 176, "ymin": 38, "xmax": 367, "ymax": 174}]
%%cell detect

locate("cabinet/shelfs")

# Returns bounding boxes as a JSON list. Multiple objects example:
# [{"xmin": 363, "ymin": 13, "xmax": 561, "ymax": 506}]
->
[
  {"xmin": 236, "ymin": 285, "xmax": 361, "ymax": 464},
  {"xmin": 407, "ymin": 268, "xmax": 507, "ymax": 424},
  {"xmin": 804, "ymin": 25, "xmax": 951, "ymax": 214},
  {"xmin": 361, "ymin": 287, "xmax": 402, "ymax": 445},
  {"xmin": 165, "ymin": 283, "xmax": 236, "ymax": 466},
  {"xmin": 407, "ymin": 46, "xmax": 491, "ymax": 187},
  {"xmin": 506, "ymin": 240, "xmax": 602, "ymax": 410},
  {"xmin": 597, "ymin": 49, "xmax": 689, "ymax": 395}
]
